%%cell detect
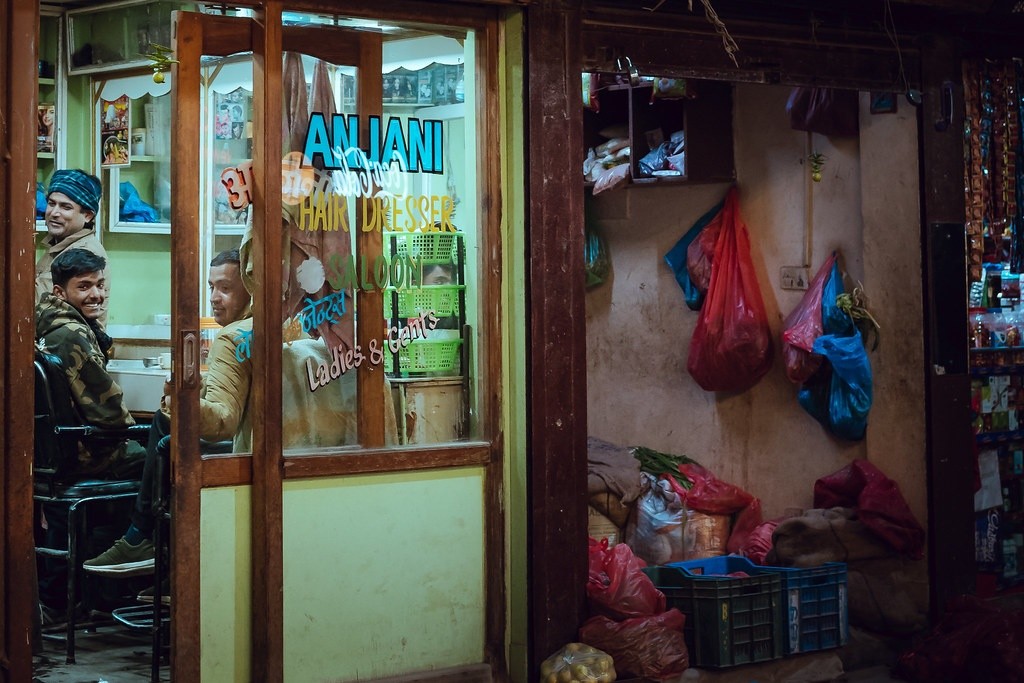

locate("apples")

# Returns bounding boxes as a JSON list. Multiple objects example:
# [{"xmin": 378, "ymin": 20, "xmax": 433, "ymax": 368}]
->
[{"xmin": 540, "ymin": 644, "xmax": 616, "ymax": 683}]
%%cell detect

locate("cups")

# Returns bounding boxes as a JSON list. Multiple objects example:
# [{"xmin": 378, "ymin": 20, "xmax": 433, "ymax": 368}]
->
[
  {"xmin": 154, "ymin": 314, "xmax": 171, "ymax": 326},
  {"xmin": 158, "ymin": 353, "xmax": 172, "ymax": 370}
]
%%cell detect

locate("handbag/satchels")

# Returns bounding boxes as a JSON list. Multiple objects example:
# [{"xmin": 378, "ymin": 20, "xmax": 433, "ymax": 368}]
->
[
  {"xmin": 540, "ymin": 643, "xmax": 617, "ymax": 683},
  {"xmin": 580, "ymin": 608, "xmax": 689, "ymax": 683},
  {"xmin": 685, "ymin": 189, "xmax": 872, "ymax": 445},
  {"xmin": 583, "ymin": 536, "xmax": 667, "ymax": 619}
]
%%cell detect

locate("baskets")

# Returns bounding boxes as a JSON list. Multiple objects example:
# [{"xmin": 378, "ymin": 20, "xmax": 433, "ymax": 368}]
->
[
  {"xmin": 383, "ymin": 284, "xmax": 466, "ymax": 316},
  {"xmin": 383, "ymin": 230, "xmax": 466, "ymax": 265},
  {"xmin": 383, "ymin": 338, "xmax": 463, "ymax": 372}
]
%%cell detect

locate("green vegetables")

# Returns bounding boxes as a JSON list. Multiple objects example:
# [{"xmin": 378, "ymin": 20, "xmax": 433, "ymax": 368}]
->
[{"xmin": 634, "ymin": 447, "xmax": 701, "ymax": 491}]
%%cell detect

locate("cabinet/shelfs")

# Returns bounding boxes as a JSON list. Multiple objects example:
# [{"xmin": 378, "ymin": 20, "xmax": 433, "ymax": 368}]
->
[
  {"xmin": 582, "ymin": 78, "xmax": 737, "ymax": 188},
  {"xmin": 109, "ymin": 53, "xmax": 342, "ymax": 234},
  {"xmin": 35, "ymin": 5, "xmax": 69, "ymax": 231}
]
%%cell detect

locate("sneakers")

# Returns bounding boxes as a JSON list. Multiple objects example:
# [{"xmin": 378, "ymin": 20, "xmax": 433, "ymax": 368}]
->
[
  {"xmin": 83, "ymin": 536, "xmax": 155, "ymax": 573},
  {"xmin": 137, "ymin": 577, "xmax": 171, "ymax": 602}
]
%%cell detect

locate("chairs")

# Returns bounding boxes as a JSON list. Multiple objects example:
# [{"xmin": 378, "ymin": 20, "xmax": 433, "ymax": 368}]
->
[{"xmin": 32, "ymin": 352, "xmax": 233, "ymax": 683}]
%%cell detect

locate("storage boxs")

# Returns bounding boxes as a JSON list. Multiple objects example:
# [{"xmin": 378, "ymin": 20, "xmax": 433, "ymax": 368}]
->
[{"xmin": 641, "ymin": 553, "xmax": 849, "ymax": 669}]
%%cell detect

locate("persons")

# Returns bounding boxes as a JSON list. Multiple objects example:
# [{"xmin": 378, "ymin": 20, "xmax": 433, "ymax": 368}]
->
[
  {"xmin": 420, "ymin": 78, "xmax": 455, "ymax": 98},
  {"xmin": 382, "ymin": 75, "xmax": 414, "ymax": 99},
  {"xmin": 412, "ymin": 264, "xmax": 458, "ymax": 330},
  {"xmin": 84, "ymin": 248, "xmax": 253, "ymax": 606},
  {"xmin": 214, "ymin": 90, "xmax": 243, "ymax": 139},
  {"xmin": 38, "ymin": 102, "xmax": 54, "ymax": 139},
  {"xmin": 33, "ymin": 248, "xmax": 146, "ymax": 475},
  {"xmin": 36, "ymin": 169, "xmax": 108, "ymax": 334}
]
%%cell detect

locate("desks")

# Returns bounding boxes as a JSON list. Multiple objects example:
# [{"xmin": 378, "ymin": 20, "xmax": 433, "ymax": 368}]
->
[{"xmin": 104, "ymin": 360, "xmax": 467, "ymax": 446}]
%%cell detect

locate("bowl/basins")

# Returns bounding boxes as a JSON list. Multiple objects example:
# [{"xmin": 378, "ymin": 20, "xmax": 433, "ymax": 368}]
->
[{"xmin": 143, "ymin": 357, "xmax": 158, "ymax": 368}]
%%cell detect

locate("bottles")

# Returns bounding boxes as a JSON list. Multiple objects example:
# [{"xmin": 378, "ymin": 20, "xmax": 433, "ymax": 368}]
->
[
  {"xmin": 130, "ymin": 127, "xmax": 146, "ymax": 156},
  {"xmin": 968, "ymin": 273, "xmax": 1024, "ymax": 349},
  {"xmin": 199, "ymin": 316, "xmax": 224, "ymax": 370}
]
